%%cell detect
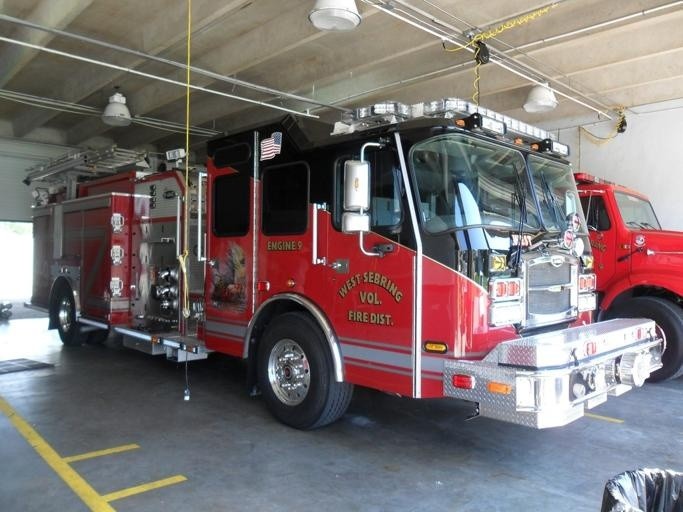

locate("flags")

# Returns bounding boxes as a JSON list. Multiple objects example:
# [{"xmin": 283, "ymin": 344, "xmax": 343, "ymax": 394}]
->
[{"xmin": 260, "ymin": 132, "xmax": 283, "ymax": 162}]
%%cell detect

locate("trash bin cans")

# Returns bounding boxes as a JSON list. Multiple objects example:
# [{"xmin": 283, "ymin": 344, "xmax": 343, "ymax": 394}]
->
[{"xmin": 600, "ymin": 468, "xmax": 683, "ymax": 512}]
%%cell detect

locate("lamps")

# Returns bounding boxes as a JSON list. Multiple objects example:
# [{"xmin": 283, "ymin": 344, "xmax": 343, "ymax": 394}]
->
[
  {"xmin": 521, "ymin": 78, "xmax": 559, "ymax": 116},
  {"xmin": 98, "ymin": 83, "xmax": 133, "ymax": 129},
  {"xmin": 307, "ymin": 0, "xmax": 361, "ymax": 35}
]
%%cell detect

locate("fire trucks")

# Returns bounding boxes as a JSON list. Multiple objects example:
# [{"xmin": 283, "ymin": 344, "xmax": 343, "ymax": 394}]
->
[
  {"xmin": 572, "ymin": 172, "xmax": 683, "ymax": 386},
  {"xmin": 21, "ymin": 95, "xmax": 666, "ymax": 430}
]
